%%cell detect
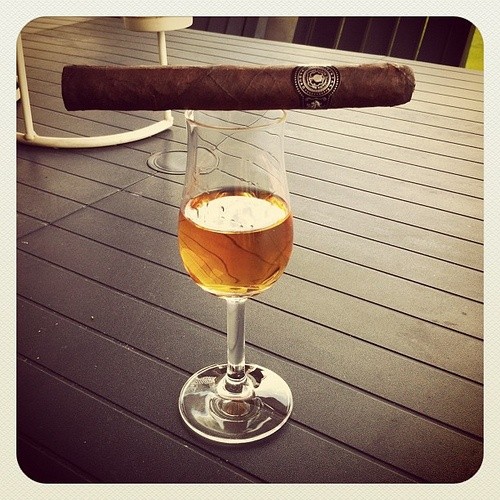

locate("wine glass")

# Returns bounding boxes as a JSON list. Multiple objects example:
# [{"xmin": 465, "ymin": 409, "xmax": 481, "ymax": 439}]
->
[{"xmin": 176, "ymin": 109, "xmax": 294, "ymax": 443}]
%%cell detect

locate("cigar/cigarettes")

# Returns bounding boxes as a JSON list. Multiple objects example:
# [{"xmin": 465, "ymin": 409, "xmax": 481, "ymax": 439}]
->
[{"xmin": 60, "ymin": 61, "xmax": 415, "ymax": 112}]
